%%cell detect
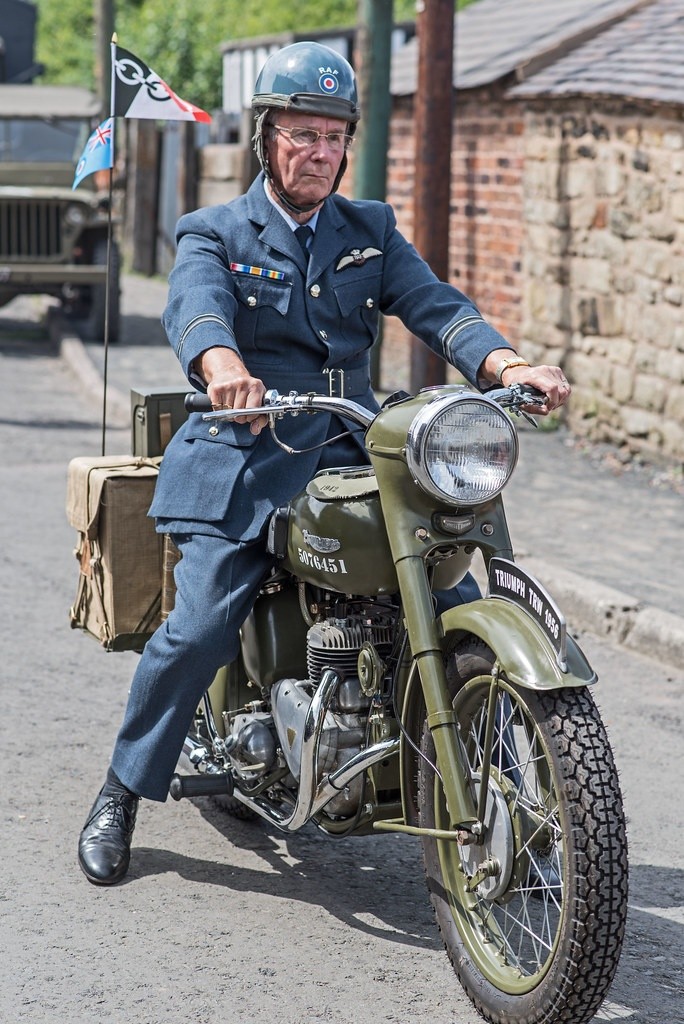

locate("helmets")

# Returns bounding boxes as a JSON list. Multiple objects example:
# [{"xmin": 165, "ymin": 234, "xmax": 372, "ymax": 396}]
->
[{"xmin": 251, "ymin": 41, "xmax": 357, "ymax": 121}]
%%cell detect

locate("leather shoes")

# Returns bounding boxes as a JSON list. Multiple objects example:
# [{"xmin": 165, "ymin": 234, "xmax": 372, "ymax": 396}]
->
[
  {"xmin": 77, "ymin": 780, "xmax": 140, "ymax": 885},
  {"xmin": 523, "ymin": 858, "xmax": 561, "ymax": 902}
]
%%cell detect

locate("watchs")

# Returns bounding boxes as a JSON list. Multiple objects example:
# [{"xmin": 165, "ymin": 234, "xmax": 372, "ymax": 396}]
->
[{"xmin": 494, "ymin": 356, "xmax": 531, "ymax": 383}]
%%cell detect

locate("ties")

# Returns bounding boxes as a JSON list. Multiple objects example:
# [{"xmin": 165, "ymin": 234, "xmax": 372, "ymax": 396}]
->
[{"xmin": 294, "ymin": 226, "xmax": 312, "ymax": 264}]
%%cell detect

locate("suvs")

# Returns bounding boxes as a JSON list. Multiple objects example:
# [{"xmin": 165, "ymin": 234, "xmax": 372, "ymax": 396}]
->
[{"xmin": 0, "ymin": 82, "xmax": 123, "ymax": 347}]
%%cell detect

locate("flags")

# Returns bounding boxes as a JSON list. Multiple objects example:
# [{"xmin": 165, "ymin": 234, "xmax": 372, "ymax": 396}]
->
[
  {"xmin": 72, "ymin": 116, "xmax": 114, "ymax": 190},
  {"xmin": 113, "ymin": 44, "xmax": 213, "ymax": 123}
]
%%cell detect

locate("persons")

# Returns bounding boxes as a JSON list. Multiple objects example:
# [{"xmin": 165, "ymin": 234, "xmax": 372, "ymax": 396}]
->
[{"xmin": 75, "ymin": 41, "xmax": 572, "ymax": 886}]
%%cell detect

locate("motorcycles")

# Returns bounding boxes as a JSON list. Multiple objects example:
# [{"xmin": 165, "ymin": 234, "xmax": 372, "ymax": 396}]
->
[{"xmin": 120, "ymin": 381, "xmax": 632, "ymax": 1024}]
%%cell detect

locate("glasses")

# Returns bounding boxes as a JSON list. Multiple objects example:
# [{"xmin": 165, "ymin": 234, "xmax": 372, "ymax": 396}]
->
[{"xmin": 265, "ymin": 121, "xmax": 356, "ymax": 152}]
